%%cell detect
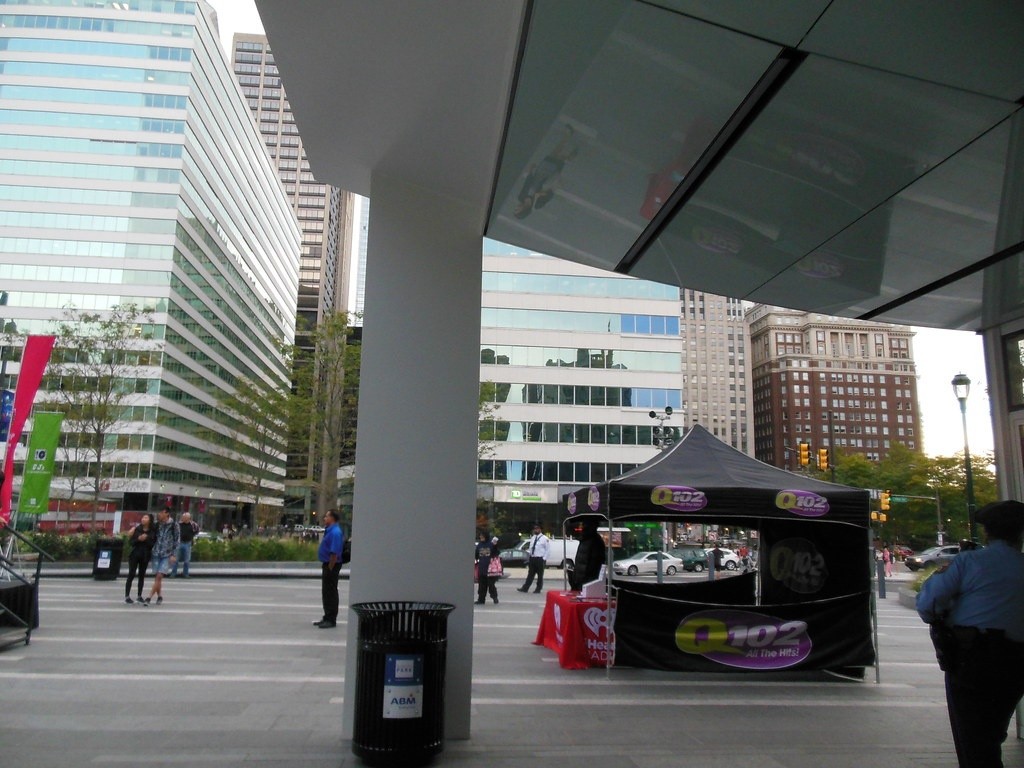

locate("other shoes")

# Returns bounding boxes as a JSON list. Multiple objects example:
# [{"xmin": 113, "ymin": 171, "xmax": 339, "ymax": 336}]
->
[
  {"xmin": 137, "ymin": 597, "xmax": 144, "ymax": 603},
  {"xmin": 144, "ymin": 598, "xmax": 151, "ymax": 606},
  {"xmin": 314, "ymin": 616, "xmax": 325, "ymax": 625},
  {"xmin": 494, "ymin": 598, "xmax": 498, "ymax": 604},
  {"xmin": 156, "ymin": 598, "xmax": 162, "ymax": 605},
  {"xmin": 518, "ymin": 589, "xmax": 528, "ymax": 592},
  {"xmin": 475, "ymin": 599, "xmax": 485, "ymax": 604},
  {"xmin": 533, "ymin": 589, "xmax": 541, "ymax": 593},
  {"xmin": 320, "ymin": 621, "xmax": 336, "ymax": 628},
  {"xmin": 126, "ymin": 598, "xmax": 133, "ymax": 604}
]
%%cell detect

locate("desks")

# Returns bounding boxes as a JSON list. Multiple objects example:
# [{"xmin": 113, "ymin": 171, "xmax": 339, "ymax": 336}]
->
[{"xmin": 529, "ymin": 590, "xmax": 616, "ymax": 670}]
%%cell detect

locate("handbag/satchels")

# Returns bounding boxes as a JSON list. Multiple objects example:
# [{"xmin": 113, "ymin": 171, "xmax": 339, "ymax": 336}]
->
[
  {"xmin": 342, "ymin": 542, "xmax": 351, "ymax": 563},
  {"xmin": 488, "ymin": 556, "xmax": 503, "ymax": 576},
  {"xmin": 475, "ymin": 562, "xmax": 480, "ymax": 583}
]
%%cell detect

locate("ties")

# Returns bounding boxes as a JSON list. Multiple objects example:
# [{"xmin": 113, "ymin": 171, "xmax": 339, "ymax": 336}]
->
[{"xmin": 532, "ymin": 535, "xmax": 542, "ymax": 554}]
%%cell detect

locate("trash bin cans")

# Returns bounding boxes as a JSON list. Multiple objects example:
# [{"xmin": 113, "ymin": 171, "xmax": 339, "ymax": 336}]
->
[
  {"xmin": 92, "ymin": 539, "xmax": 125, "ymax": 580},
  {"xmin": 348, "ymin": 601, "xmax": 457, "ymax": 763}
]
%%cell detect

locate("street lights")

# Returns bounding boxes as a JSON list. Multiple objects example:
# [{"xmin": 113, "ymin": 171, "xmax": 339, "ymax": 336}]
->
[
  {"xmin": 950, "ymin": 373, "xmax": 979, "ymax": 544},
  {"xmin": 646, "ymin": 404, "xmax": 678, "ymax": 584}
]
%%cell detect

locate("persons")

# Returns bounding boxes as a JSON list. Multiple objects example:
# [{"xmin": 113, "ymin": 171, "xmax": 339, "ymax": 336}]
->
[
  {"xmin": 222, "ymin": 519, "xmax": 322, "ymax": 545},
  {"xmin": 712, "ymin": 542, "xmax": 725, "ymax": 581},
  {"xmin": 144, "ymin": 507, "xmax": 179, "ymax": 606},
  {"xmin": 914, "ymin": 498, "xmax": 1024, "ymax": 768},
  {"xmin": 517, "ymin": 521, "xmax": 551, "ymax": 595},
  {"xmin": 568, "ymin": 517, "xmax": 605, "ymax": 591},
  {"xmin": 734, "ymin": 544, "xmax": 748, "ymax": 567},
  {"xmin": 510, "ymin": 124, "xmax": 588, "ymax": 221},
  {"xmin": 312, "ymin": 507, "xmax": 344, "ymax": 630},
  {"xmin": 882, "ymin": 545, "xmax": 894, "ymax": 581},
  {"xmin": 171, "ymin": 510, "xmax": 200, "ymax": 581},
  {"xmin": 474, "ymin": 530, "xmax": 503, "ymax": 606},
  {"xmin": 36, "ymin": 523, "xmax": 106, "ymax": 535},
  {"xmin": 124, "ymin": 513, "xmax": 155, "ymax": 604}
]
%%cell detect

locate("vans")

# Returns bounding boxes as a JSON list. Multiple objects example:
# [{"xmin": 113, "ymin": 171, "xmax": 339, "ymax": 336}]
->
[
  {"xmin": 514, "ymin": 539, "xmax": 580, "ymax": 570},
  {"xmin": 286, "ymin": 524, "xmax": 305, "ymax": 532}
]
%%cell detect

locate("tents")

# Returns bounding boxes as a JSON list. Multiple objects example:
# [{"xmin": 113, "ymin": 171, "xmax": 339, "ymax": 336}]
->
[{"xmin": 563, "ymin": 424, "xmax": 875, "ymax": 686}]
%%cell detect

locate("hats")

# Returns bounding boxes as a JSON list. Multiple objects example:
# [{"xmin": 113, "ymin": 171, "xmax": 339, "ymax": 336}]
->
[{"xmin": 975, "ymin": 498, "xmax": 1024, "ymax": 526}]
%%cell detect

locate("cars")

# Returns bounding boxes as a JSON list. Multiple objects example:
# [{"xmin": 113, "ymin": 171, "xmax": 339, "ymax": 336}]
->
[
  {"xmin": 613, "ymin": 551, "xmax": 684, "ymax": 575},
  {"xmin": 305, "ymin": 525, "xmax": 325, "ymax": 533},
  {"xmin": 903, "ymin": 545, "xmax": 961, "ymax": 572},
  {"xmin": 667, "ymin": 548, "xmax": 709, "ymax": 572},
  {"xmin": 700, "ymin": 548, "xmax": 740, "ymax": 570},
  {"xmin": 497, "ymin": 550, "xmax": 532, "ymax": 568}
]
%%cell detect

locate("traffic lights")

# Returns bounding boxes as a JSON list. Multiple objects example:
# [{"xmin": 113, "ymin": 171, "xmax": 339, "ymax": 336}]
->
[
  {"xmin": 312, "ymin": 512, "xmax": 317, "ymax": 517},
  {"xmin": 871, "ymin": 512, "xmax": 879, "ymax": 520},
  {"xmin": 880, "ymin": 515, "xmax": 887, "ymax": 521},
  {"xmin": 800, "ymin": 441, "xmax": 810, "ymax": 466},
  {"xmin": 881, "ymin": 493, "xmax": 891, "ymax": 511},
  {"xmin": 819, "ymin": 448, "xmax": 829, "ymax": 471}
]
